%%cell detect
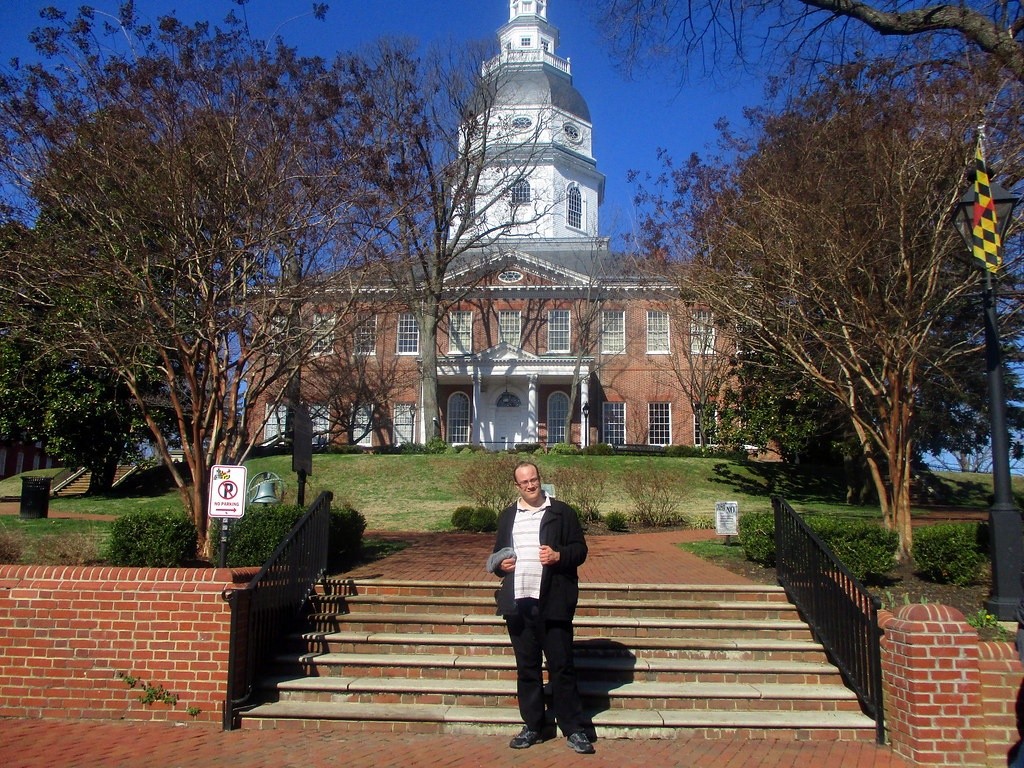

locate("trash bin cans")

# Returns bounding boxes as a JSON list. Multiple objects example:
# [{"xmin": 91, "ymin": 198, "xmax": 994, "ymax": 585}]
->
[{"xmin": 19, "ymin": 475, "xmax": 55, "ymax": 520}]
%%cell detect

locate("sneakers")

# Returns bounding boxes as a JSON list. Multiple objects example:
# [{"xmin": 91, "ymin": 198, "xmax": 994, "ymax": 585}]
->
[
  {"xmin": 509, "ymin": 726, "xmax": 545, "ymax": 749},
  {"xmin": 566, "ymin": 731, "xmax": 595, "ymax": 754}
]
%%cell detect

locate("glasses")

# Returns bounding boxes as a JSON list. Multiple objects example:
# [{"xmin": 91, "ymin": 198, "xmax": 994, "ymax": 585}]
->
[{"xmin": 517, "ymin": 477, "xmax": 539, "ymax": 486}]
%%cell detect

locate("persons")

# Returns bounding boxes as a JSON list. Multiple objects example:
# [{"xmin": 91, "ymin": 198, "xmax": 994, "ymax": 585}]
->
[{"xmin": 494, "ymin": 461, "xmax": 595, "ymax": 754}]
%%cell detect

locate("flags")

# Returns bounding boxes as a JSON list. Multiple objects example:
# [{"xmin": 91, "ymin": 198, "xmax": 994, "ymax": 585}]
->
[{"xmin": 972, "ymin": 135, "xmax": 1004, "ymax": 272}]
